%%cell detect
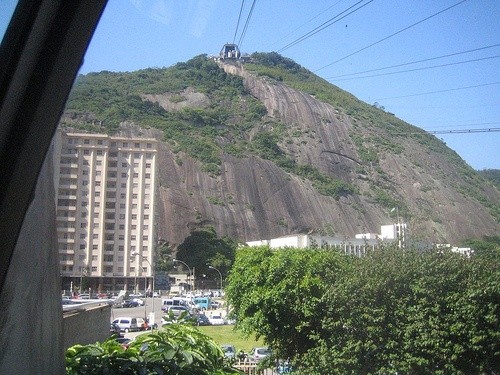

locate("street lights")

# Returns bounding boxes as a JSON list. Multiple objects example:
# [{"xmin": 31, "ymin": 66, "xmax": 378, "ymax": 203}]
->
[
  {"xmin": 134, "ymin": 252, "xmax": 155, "ymax": 313},
  {"xmin": 173, "ymin": 258, "xmax": 192, "ymax": 295},
  {"xmin": 209, "ymin": 266, "xmax": 223, "ymax": 297}
]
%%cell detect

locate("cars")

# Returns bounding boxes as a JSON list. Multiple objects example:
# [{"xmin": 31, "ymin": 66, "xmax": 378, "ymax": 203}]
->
[{"xmin": 59, "ymin": 285, "xmax": 276, "ymax": 365}]
